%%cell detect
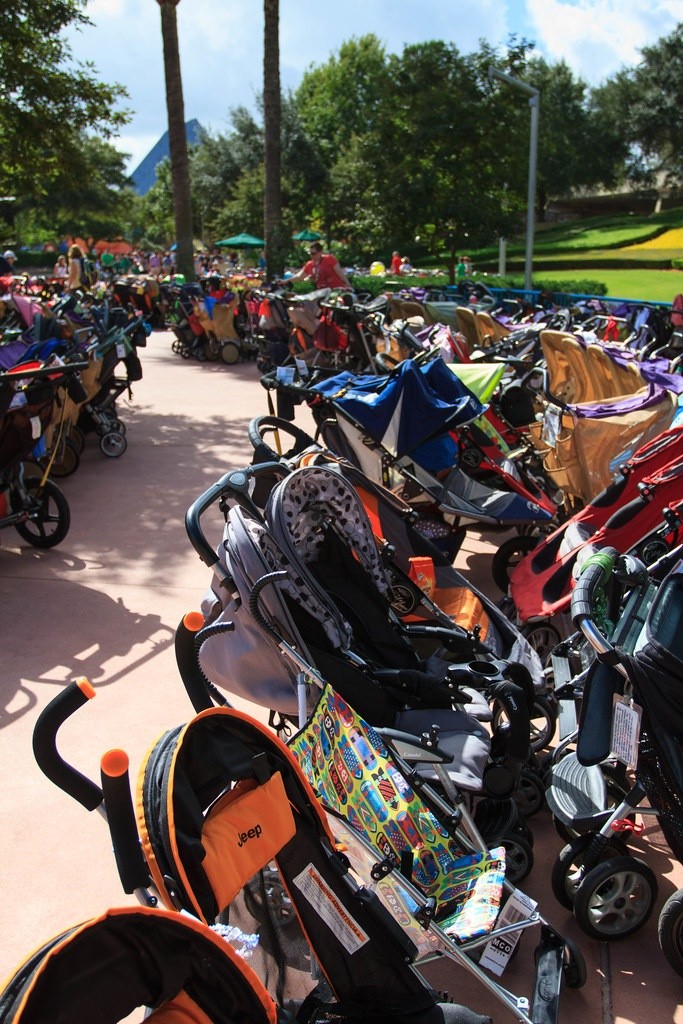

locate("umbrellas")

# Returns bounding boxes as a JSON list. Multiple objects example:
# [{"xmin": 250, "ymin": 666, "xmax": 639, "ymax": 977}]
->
[
  {"xmin": 214, "ymin": 233, "xmax": 267, "ymax": 250},
  {"xmin": 289, "ymin": 227, "xmax": 322, "ymax": 242}
]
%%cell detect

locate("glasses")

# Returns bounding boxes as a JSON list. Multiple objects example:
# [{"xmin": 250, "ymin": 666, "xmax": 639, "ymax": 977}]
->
[{"xmin": 311, "ymin": 251, "xmax": 319, "ymax": 256}]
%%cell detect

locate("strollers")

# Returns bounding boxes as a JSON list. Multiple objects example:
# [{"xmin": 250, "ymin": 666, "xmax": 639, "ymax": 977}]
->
[
  {"xmin": 540, "ymin": 545, "xmax": 682, "ymax": 960},
  {"xmin": 191, "ymin": 569, "xmax": 588, "ymax": 1024},
  {"xmin": 32, "ymin": 610, "xmax": 495, "ymax": 1024},
  {"xmin": 0, "ymin": 905, "xmax": 283, "ymax": 1024},
  {"xmin": 0, "ymin": 266, "xmax": 683, "ymax": 1024}
]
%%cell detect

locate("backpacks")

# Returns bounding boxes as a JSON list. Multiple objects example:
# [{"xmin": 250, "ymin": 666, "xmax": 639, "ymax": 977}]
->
[{"xmin": 79, "ymin": 257, "xmax": 99, "ymax": 287}]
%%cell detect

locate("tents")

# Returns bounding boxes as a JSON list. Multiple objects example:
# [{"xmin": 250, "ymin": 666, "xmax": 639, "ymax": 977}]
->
[{"xmin": 19, "ymin": 233, "xmax": 208, "ymax": 255}]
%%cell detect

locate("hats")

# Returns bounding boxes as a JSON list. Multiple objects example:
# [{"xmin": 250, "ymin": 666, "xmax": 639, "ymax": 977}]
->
[
  {"xmin": 401, "ymin": 256, "xmax": 412, "ymax": 263},
  {"xmin": 4, "ymin": 250, "xmax": 18, "ymax": 261},
  {"xmin": 463, "ymin": 256, "xmax": 472, "ymax": 261}
]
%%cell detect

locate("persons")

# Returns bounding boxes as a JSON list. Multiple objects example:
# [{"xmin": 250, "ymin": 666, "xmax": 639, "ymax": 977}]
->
[
  {"xmin": 258, "ymin": 252, "xmax": 265, "ymax": 273},
  {"xmin": 87, "ymin": 247, "xmax": 243, "ymax": 275},
  {"xmin": 275, "ymin": 242, "xmax": 351, "ymax": 290},
  {"xmin": 390, "ymin": 252, "xmax": 412, "ymax": 276},
  {"xmin": 0, "ymin": 250, "xmax": 18, "ymax": 301},
  {"xmin": 54, "ymin": 244, "xmax": 85, "ymax": 291},
  {"xmin": 454, "ymin": 257, "xmax": 471, "ymax": 277}
]
